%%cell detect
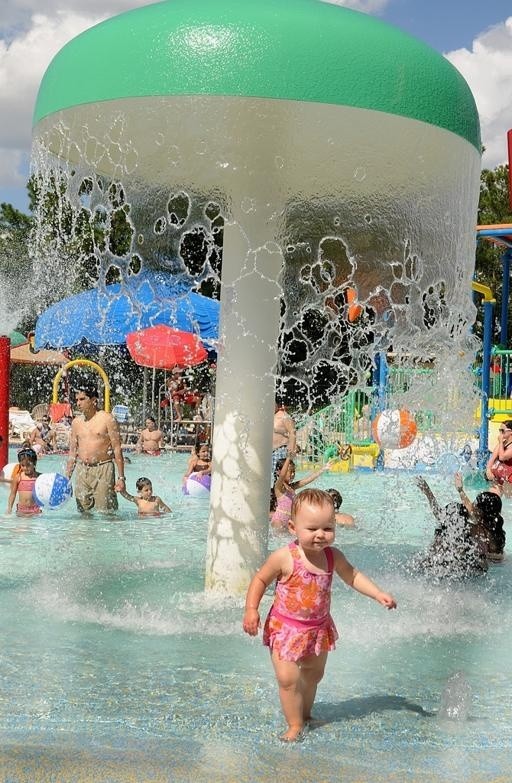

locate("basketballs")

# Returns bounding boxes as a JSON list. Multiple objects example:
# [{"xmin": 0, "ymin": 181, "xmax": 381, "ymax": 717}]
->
[
  {"xmin": 33, "ymin": 473, "xmax": 70, "ymax": 509},
  {"xmin": 373, "ymin": 409, "xmax": 416, "ymax": 449},
  {"xmin": 182, "ymin": 472, "xmax": 210, "ymax": 497}
]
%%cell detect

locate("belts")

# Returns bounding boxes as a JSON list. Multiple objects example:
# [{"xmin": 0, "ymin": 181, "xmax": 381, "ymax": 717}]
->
[{"xmin": 83, "ymin": 459, "xmax": 112, "ymax": 469}]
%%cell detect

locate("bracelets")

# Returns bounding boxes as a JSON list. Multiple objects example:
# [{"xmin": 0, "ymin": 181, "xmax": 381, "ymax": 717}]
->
[{"xmin": 456, "ymin": 486, "xmax": 464, "ymax": 492}]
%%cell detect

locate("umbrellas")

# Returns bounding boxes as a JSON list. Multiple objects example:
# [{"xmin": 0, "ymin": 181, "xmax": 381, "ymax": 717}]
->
[
  {"xmin": 37, "ymin": 267, "xmax": 220, "ymax": 430},
  {"xmin": 125, "ymin": 321, "xmax": 209, "ymax": 369}
]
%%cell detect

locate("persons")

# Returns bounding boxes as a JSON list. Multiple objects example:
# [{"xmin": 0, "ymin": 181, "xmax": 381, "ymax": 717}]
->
[
  {"xmin": 411, "ymin": 475, "xmax": 489, "ymax": 578},
  {"xmin": 4, "ymin": 368, "xmax": 213, "ymax": 523},
  {"xmin": 326, "ymin": 491, "xmax": 353, "ymax": 527},
  {"xmin": 485, "ymin": 419, "xmax": 512, "ymax": 498},
  {"xmin": 271, "ymin": 439, "xmax": 333, "ymax": 532},
  {"xmin": 454, "ymin": 473, "xmax": 507, "ymax": 563},
  {"xmin": 269, "ymin": 392, "xmax": 374, "ymax": 472},
  {"xmin": 244, "ymin": 487, "xmax": 399, "ymax": 739}
]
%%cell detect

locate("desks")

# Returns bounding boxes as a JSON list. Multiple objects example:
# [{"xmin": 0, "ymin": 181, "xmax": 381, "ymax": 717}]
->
[{"xmin": 162, "ymin": 419, "xmax": 212, "ymax": 446}]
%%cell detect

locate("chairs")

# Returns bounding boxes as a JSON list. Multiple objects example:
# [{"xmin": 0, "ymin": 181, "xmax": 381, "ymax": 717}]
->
[{"xmin": 9, "ymin": 404, "xmax": 134, "ymax": 443}]
